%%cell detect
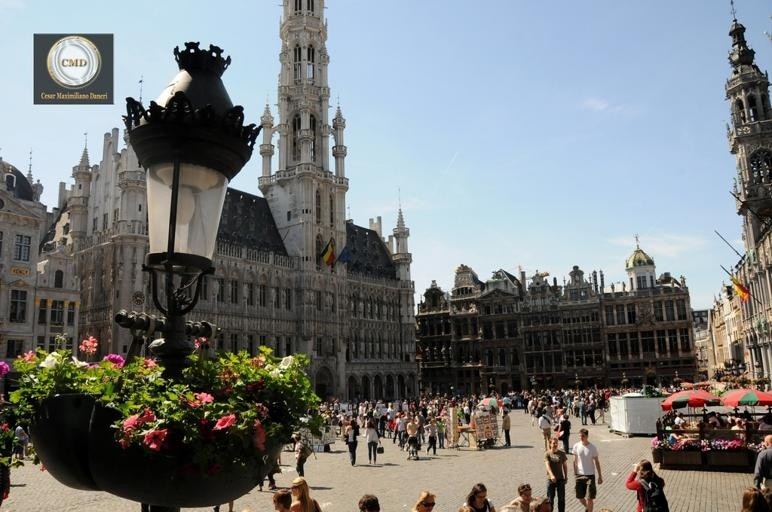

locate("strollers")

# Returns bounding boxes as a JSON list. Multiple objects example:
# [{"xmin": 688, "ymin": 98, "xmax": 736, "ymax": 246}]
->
[{"xmin": 403, "ymin": 434, "xmax": 421, "ymax": 461}]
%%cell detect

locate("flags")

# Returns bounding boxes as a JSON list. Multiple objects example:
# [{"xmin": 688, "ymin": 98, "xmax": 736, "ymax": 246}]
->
[{"xmin": 319, "ymin": 235, "xmax": 335, "ymax": 266}]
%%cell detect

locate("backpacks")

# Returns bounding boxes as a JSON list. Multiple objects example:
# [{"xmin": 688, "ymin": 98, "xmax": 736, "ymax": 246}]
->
[{"xmin": 639, "ymin": 479, "xmax": 669, "ymax": 512}]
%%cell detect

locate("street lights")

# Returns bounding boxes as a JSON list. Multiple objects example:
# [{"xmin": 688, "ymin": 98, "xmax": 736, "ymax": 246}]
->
[
  {"xmin": 622, "ymin": 372, "xmax": 628, "ymax": 390},
  {"xmin": 674, "ymin": 370, "xmax": 679, "ymax": 387},
  {"xmin": 488, "ymin": 378, "xmax": 495, "ymax": 391},
  {"xmin": 125, "ymin": 38, "xmax": 265, "ymax": 512},
  {"xmin": 574, "ymin": 374, "xmax": 582, "ymax": 390},
  {"xmin": 530, "ymin": 375, "xmax": 536, "ymax": 389},
  {"xmin": 450, "ymin": 385, "xmax": 455, "ymax": 396}
]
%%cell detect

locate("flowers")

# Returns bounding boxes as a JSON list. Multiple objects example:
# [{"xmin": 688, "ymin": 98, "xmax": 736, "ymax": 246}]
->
[{"xmin": 0, "ymin": 329, "xmax": 329, "ymax": 470}]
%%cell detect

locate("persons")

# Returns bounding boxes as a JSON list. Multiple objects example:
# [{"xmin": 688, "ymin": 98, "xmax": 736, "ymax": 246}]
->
[
  {"xmin": 523, "ymin": 387, "xmax": 664, "ymax": 424},
  {"xmin": 528, "ymin": 495, "xmax": 552, "ymax": 512},
  {"xmin": 653, "ymin": 384, "xmax": 772, "ymax": 444},
  {"xmin": 314, "ymin": 385, "xmax": 519, "ymax": 466},
  {"xmin": 358, "ymin": 494, "xmax": 380, "ymax": 512},
  {"xmin": 213, "ymin": 500, "xmax": 236, "ymax": 512},
  {"xmin": 272, "ymin": 488, "xmax": 293, "ymax": 512},
  {"xmin": 412, "ymin": 489, "xmax": 436, "ymax": 512},
  {"xmin": 543, "ymin": 437, "xmax": 568, "ymax": 512},
  {"xmin": 511, "ymin": 482, "xmax": 535, "ymax": 512},
  {"xmin": 12, "ymin": 425, "xmax": 28, "ymax": 460},
  {"xmin": 290, "ymin": 476, "xmax": 323, "ymax": 512},
  {"xmin": 752, "ymin": 434, "xmax": 772, "ymax": 494},
  {"xmin": 741, "ymin": 487, "xmax": 769, "ymax": 512},
  {"xmin": 291, "ymin": 431, "xmax": 307, "ymax": 477},
  {"xmin": 572, "ymin": 428, "xmax": 603, "ymax": 512},
  {"xmin": 457, "ymin": 483, "xmax": 496, "ymax": 512},
  {"xmin": 625, "ymin": 459, "xmax": 669, "ymax": 512},
  {"xmin": 257, "ymin": 455, "xmax": 281, "ymax": 491}
]
